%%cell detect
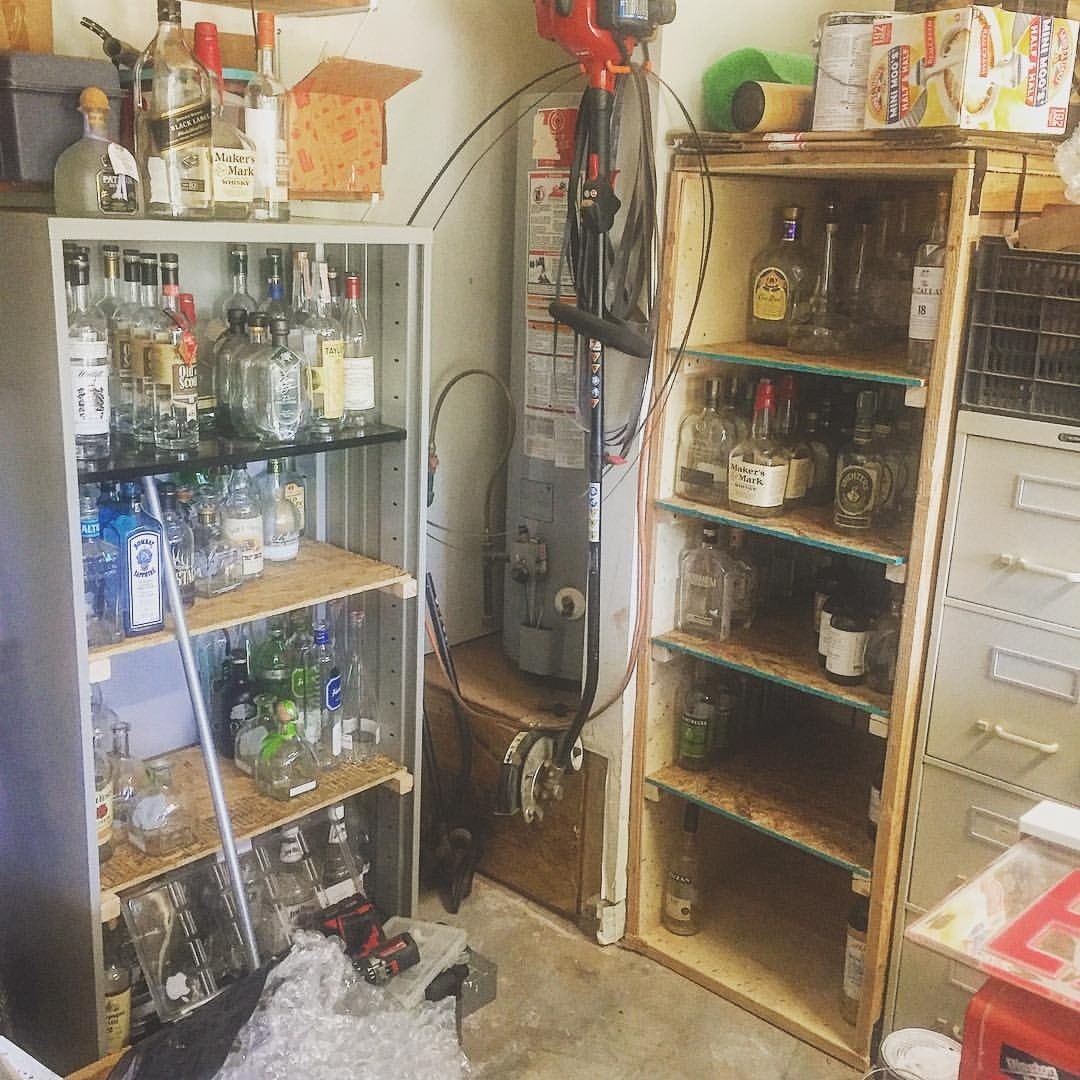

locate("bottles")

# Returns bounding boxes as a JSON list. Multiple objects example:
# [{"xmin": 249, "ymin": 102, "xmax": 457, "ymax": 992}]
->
[
  {"xmin": 201, "ymin": 458, "xmax": 307, "ymax": 579},
  {"xmin": 194, "ymin": 22, "xmax": 257, "ymax": 219},
  {"xmin": 813, "ymin": 549, "xmax": 906, "ymax": 695},
  {"xmin": 750, "ymin": 199, "xmax": 854, "ymax": 356},
  {"xmin": 96, "ymin": 245, "xmax": 199, "ymax": 450},
  {"xmin": 89, "ymin": 683, "xmax": 200, "ymax": 863},
  {"xmin": 664, "ymin": 803, "xmax": 703, "ymax": 936},
  {"xmin": 155, "ymin": 463, "xmax": 244, "ymax": 611},
  {"xmin": 54, "ymin": 88, "xmax": 145, "ymax": 219},
  {"xmin": 102, "ymin": 918, "xmax": 132, "ymax": 1055},
  {"xmin": 841, "ymin": 872, "xmax": 872, "ymax": 1026},
  {"xmin": 674, "ymin": 373, "xmax": 924, "ymax": 531},
  {"xmin": 346, "ymin": 932, "xmax": 420, "ymax": 986},
  {"xmin": 867, "ymin": 740, "xmax": 887, "ymax": 841},
  {"xmin": 193, "ymin": 621, "xmax": 321, "ymax": 801},
  {"xmin": 133, "ymin": 0, "xmax": 215, "ymax": 220},
  {"xmin": 851, "ymin": 191, "xmax": 952, "ymax": 371},
  {"xmin": 79, "ymin": 479, "xmax": 164, "ymax": 648},
  {"xmin": 237, "ymin": 801, "xmax": 364, "ymax": 964},
  {"xmin": 676, "ymin": 664, "xmax": 771, "ymax": 770},
  {"xmin": 63, "ymin": 243, "xmax": 111, "ymax": 461},
  {"xmin": 276, "ymin": 595, "xmax": 382, "ymax": 772},
  {"xmin": 677, "ymin": 521, "xmax": 795, "ymax": 643},
  {"xmin": 241, "ymin": 13, "xmax": 290, "ymax": 223},
  {"xmin": 213, "ymin": 244, "xmax": 378, "ymax": 445}
]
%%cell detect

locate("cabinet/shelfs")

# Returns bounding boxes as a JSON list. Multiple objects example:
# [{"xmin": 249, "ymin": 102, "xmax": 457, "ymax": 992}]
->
[
  {"xmin": 621, "ymin": 124, "xmax": 1080, "ymax": 1080},
  {"xmin": 0, "ymin": 200, "xmax": 439, "ymax": 1080}
]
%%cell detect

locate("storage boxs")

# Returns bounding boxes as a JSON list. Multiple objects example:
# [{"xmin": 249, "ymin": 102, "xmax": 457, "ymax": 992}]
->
[
  {"xmin": 955, "ymin": 232, "xmax": 1080, "ymax": 429},
  {"xmin": 0, "ymin": 48, "xmax": 129, "ymax": 186},
  {"xmin": 286, "ymin": 56, "xmax": 425, "ymax": 203},
  {"xmin": 862, "ymin": 7, "xmax": 1080, "ymax": 138}
]
